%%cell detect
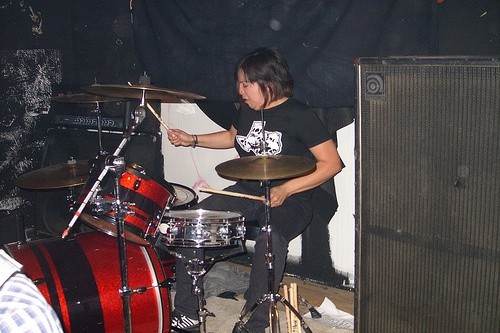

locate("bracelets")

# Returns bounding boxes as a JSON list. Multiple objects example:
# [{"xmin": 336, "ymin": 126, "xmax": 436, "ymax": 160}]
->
[{"xmin": 192, "ymin": 134, "xmax": 198, "ymax": 148}]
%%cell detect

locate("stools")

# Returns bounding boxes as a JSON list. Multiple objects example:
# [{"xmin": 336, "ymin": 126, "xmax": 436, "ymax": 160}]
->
[{"xmin": 244, "ymin": 220, "xmax": 322, "ymax": 320}]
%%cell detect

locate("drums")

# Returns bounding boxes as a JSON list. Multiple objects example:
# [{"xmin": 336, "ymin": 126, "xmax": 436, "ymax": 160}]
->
[
  {"xmin": 161, "ymin": 209, "xmax": 244, "ymax": 248},
  {"xmin": 72, "ymin": 154, "xmax": 176, "ymax": 247},
  {"xmin": 3, "ymin": 231, "xmax": 173, "ymax": 333},
  {"xmin": 166, "ymin": 182, "xmax": 199, "ymax": 210}
]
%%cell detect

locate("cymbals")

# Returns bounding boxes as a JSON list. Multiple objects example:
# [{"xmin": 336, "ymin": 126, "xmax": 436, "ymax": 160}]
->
[
  {"xmin": 83, "ymin": 85, "xmax": 205, "ymax": 100},
  {"xmin": 215, "ymin": 155, "xmax": 317, "ymax": 180},
  {"xmin": 14, "ymin": 161, "xmax": 93, "ymax": 189},
  {"xmin": 51, "ymin": 94, "xmax": 126, "ymax": 103}
]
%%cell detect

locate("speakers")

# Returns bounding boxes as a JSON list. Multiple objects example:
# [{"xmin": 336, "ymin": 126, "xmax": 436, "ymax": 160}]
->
[
  {"xmin": 355, "ymin": 56, "xmax": 500, "ymax": 333},
  {"xmin": 34, "ymin": 127, "xmax": 166, "ymax": 239}
]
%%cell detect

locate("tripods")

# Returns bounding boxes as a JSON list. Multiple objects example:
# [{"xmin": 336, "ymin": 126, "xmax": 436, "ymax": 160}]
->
[{"xmin": 233, "ymin": 179, "xmax": 313, "ymax": 333}]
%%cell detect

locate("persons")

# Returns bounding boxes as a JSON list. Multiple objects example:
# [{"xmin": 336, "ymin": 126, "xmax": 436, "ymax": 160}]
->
[{"xmin": 167, "ymin": 47, "xmax": 342, "ymax": 333}]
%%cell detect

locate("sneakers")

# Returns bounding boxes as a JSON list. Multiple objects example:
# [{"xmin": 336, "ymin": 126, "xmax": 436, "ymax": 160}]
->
[{"xmin": 170, "ymin": 310, "xmax": 199, "ymax": 332}]
